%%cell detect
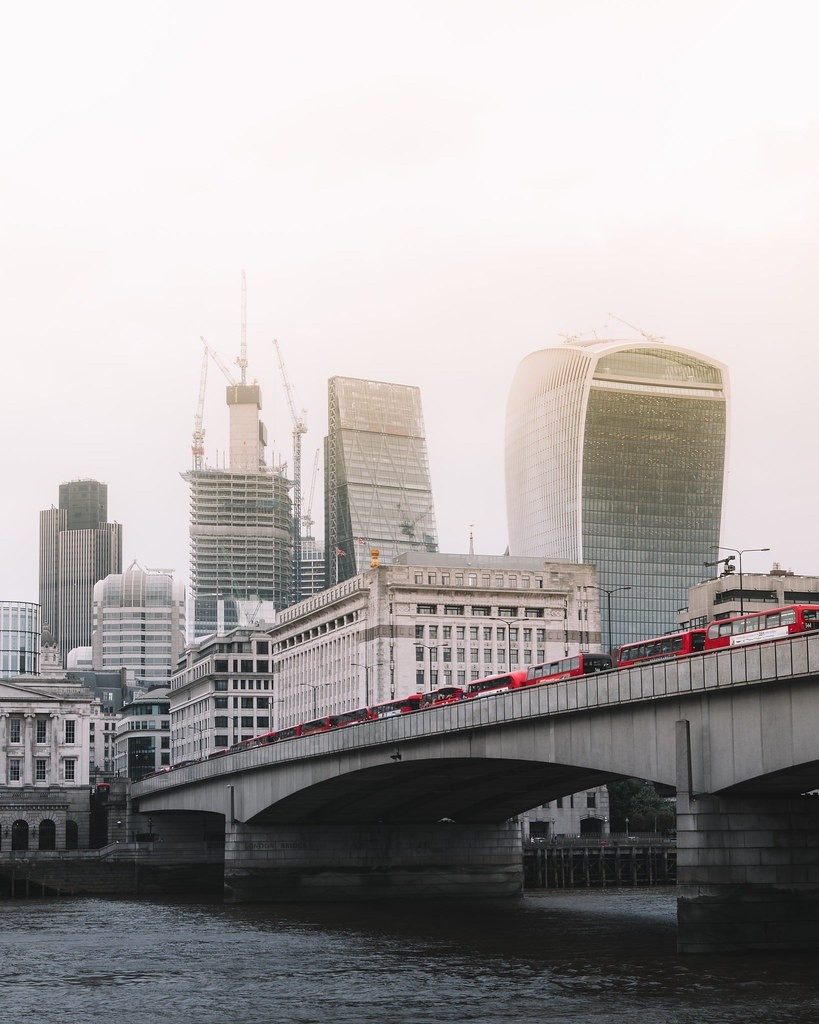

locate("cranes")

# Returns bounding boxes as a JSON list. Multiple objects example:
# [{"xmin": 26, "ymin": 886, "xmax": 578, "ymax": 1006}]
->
[{"xmin": 190, "ymin": 288, "xmax": 321, "ymax": 545}]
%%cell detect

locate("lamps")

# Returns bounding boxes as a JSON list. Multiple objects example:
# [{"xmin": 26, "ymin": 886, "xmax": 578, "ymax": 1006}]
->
[
  {"xmin": 4, "ymin": 825, "xmax": 10, "ymax": 837},
  {"xmin": 114, "ymin": 819, "xmax": 123, "ymax": 827},
  {"xmin": 30, "ymin": 826, "xmax": 38, "ymax": 838}
]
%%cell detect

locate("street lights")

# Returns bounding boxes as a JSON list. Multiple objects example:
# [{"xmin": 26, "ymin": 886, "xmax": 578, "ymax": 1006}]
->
[
  {"xmin": 189, "ymin": 726, "xmax": 214, "ymax": 757},
  {"xmin": 413, "ymin": 642, "xmax": 449, "ymax": 692},
  {"xmin": 576, "ymin": 584, "xmax": 631, "ymax": 659},
  {"xmin": 258, "ymin": 698, "xmax": 285, "ymax": 731},
  {"xmin": 300, "ymin": 682, "xmax": 331, "ymax": 719},
  {"xmin": 488, "ymin": 616, "xmax": 529, "ymax": 671},
  {"xmin": 708, "ymin": 545, "xmax": 770, "ymax": 617},
  {"xmin": 351, "ymin": 662, "xmax": 384, "ymax": 705}
]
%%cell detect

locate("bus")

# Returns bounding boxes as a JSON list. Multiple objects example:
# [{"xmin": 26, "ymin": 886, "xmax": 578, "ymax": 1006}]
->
[
  {"xmin": 417, "ymin": 687, "xmax": 463, "ymax": 709},
  {"xmin": 703, "ymin": 603, "xmax": 819, "ymax": 657},
  {"xmin": 136, "ymin": 706, "xmax": 370, "ymax": 780},
  {"xmin": 366, "ymin": 694, "xmax": 423, "ymax": 720},
  {"xmin": 617, "ymin": 629, "xmax": 707, "ymax": 668},
  {"xmin": 463, "ymin": 670, "xmax": 525, "ymax": 702},
  {"xmin": 525, "ymin": 652, "xmax": 612, "ymax": 686}
]
